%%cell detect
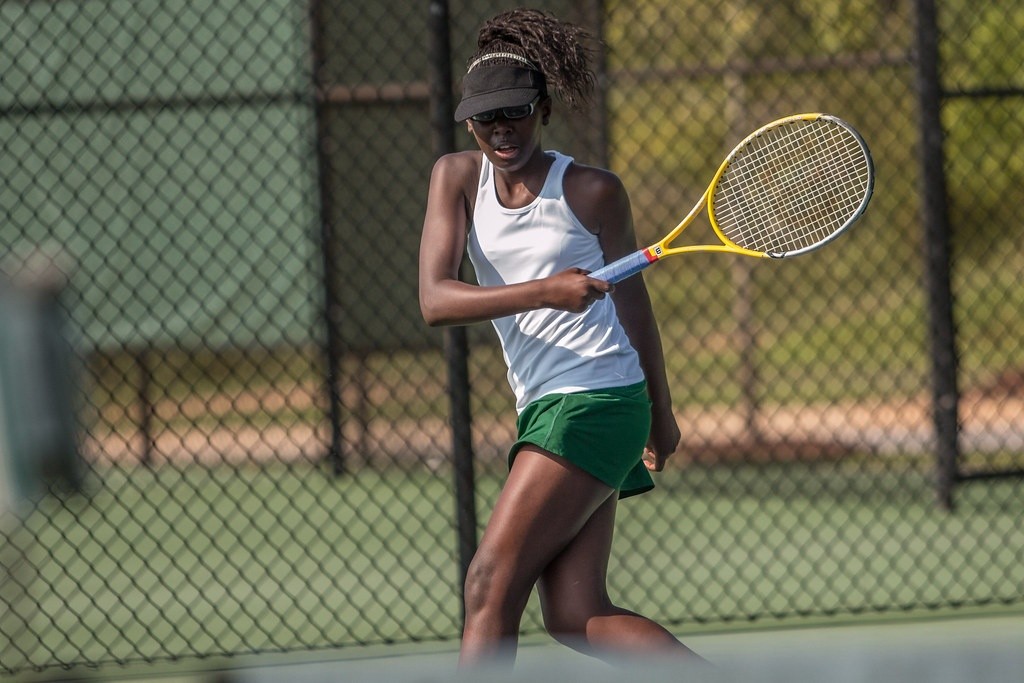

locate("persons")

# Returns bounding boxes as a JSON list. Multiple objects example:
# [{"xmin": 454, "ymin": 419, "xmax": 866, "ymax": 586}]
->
[
  {"xmin": 25, "ymin": 252, "xmax": 85, "ymax": 492},
  {"xmin": 420, "ymin": 7, "xmax": 721, "ymax": 675}
]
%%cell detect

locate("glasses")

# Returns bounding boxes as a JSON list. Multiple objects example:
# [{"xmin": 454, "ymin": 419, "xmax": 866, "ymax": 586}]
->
[{"xmin": 469, "ymin": 95, "xmax": 541, "ymax": 125}]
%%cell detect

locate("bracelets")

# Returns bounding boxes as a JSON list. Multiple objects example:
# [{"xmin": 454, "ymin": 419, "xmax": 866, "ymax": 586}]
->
[{"xmin": 652, "ymin": 396, "xmax": 670, "ymax": 416}]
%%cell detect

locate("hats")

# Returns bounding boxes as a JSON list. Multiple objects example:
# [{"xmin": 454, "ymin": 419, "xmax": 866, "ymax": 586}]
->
[{"xmin": 454, "ymin": 66, "xmax": 548, "ymax": 122}]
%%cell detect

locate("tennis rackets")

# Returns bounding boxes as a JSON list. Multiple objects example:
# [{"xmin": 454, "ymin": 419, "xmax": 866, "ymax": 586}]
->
[{"xmin": 583, "ymin": 111, "xmax": 875, "ymax": 286}]
